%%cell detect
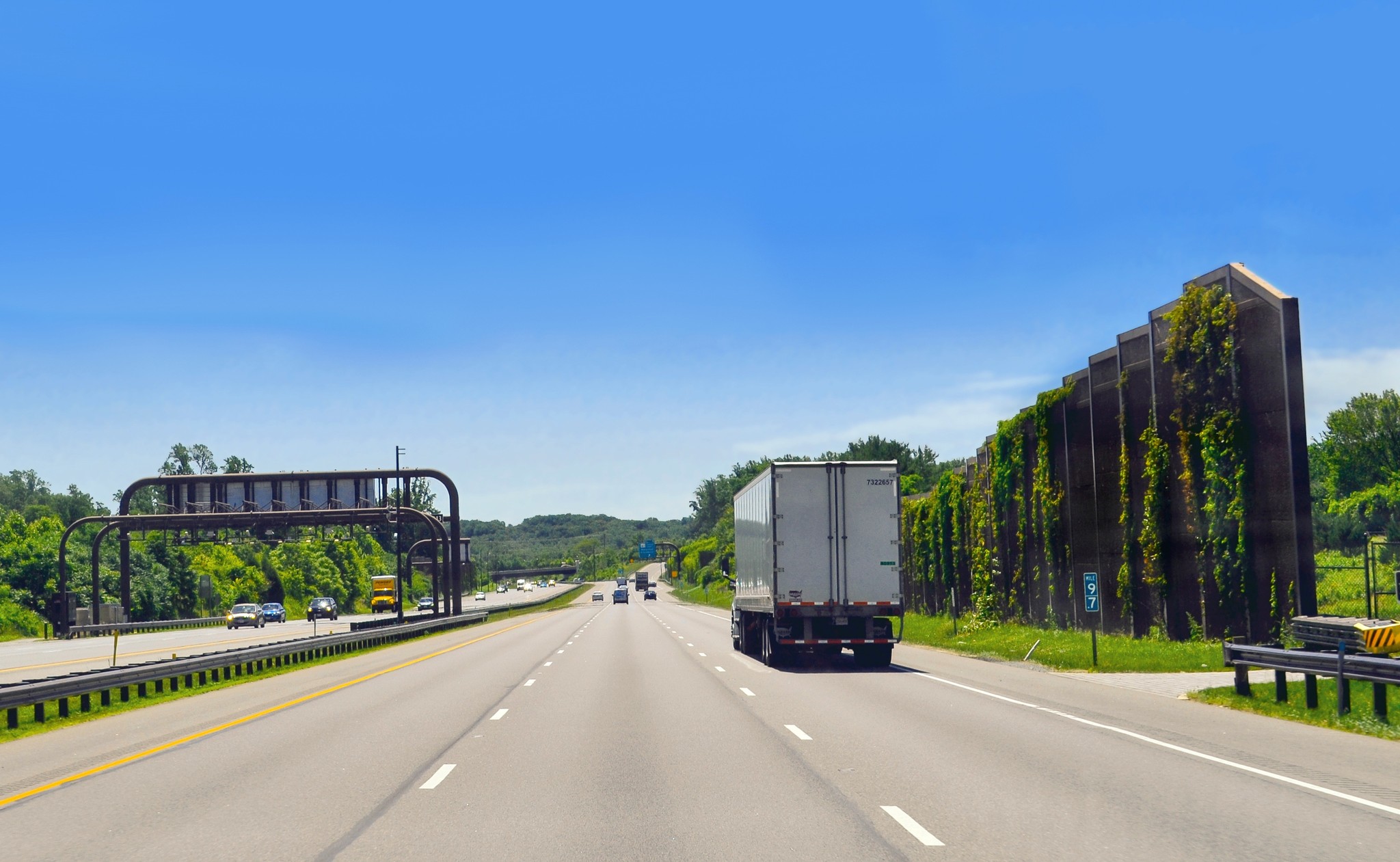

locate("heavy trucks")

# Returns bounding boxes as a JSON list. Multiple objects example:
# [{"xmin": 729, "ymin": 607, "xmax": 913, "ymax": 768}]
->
[{"xmin": 720, "ymin": 459, "xmax": 906, "ymax": 667}]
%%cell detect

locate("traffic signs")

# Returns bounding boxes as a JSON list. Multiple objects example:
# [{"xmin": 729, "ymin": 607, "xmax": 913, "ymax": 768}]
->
[{"xmin": 638, "ymin": 540, "xmax": 656, "ymax": 560}]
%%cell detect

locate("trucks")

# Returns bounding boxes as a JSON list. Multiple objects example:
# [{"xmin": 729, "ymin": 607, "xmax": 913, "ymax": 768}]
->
[
  {"xmin": 635, "ymin": 571, "xmax": 649, "ymax": 591},
  {"xmin": 616, "ymin": 577, "xmax": 627, "ymax": 587},
  {"xmin": 369, "ymin": 575, "xmax": 398, "ymax": 614}
]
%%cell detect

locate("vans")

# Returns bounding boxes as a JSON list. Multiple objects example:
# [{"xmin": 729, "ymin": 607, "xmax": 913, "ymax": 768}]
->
[{"xmin": 516, "ymin": 578, "xmax": 526, "ymax": 590}]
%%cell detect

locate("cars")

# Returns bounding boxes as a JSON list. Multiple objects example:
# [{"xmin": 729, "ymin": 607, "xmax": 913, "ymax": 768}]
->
[
  {"xmin": 417, "ymin": 598, "xmax": 435, "ymax": 611},
  {"xmin": 475, "ymin": 592, "xmax": 488, "ymax": 601},
  {"xmin": 573, "ymin": 577, "xmax": 585, "ymax": 582},
  {"xmin": 261, "ymin": 603, "xmax": 289, "ymax": 623},
  {"xmin": 496, "ymin": 585, "xmax": 510, "ymax": 593},
  {"xmin": 227, "ymin": 603, "xmax": 265, "ymax": 630},
  {"xmin": 620, "ymin": 585, "xmax": 629, "ymax": 590},
  {"xmin": 643, "ymin": 591, "xmax": 657, "ymax": 601},
  {"xmin": 524, "ymin": 583, "xmax": 533, "ymax": 593},
  {"xmin": 592, "ymin": 591, "xmax": 604, "ymax": 602},
  {"xmin": 650, "ymin": 581, "xmax": 657, "ymax": 587},
  {"xmin": 629, "ymin": 577, "xmax": 638, "ymax": 582}
]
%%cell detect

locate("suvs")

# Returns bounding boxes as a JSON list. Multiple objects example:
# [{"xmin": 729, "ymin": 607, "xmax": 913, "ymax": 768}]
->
[
  {"xmin": 537, "ymin": 579, "xmax": 556, "ymax": 588},
  {"xmin": 612, "ymin": 589, "xmax": 630, "ymax": 605},
  {"xmin": 307, "ymin": 598, "xmax": 338, "ymax": 621}
]
